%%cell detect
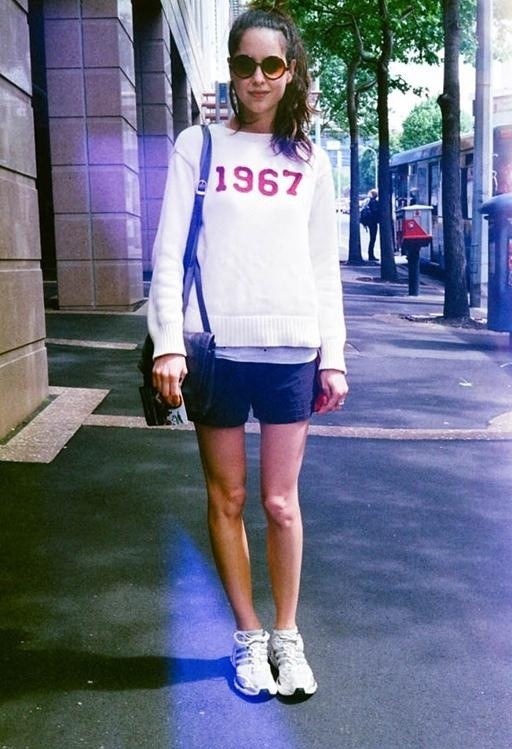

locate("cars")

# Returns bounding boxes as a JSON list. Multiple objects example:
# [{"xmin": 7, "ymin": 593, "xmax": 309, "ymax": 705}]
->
[{"xmin": 335, "ymin": 195, "xmax": 369, "ymax": 214}]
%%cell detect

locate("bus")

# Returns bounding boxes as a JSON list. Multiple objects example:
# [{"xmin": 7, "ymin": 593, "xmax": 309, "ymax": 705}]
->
[{"xmin": 388, "ymin": 122, "xmax": 512, "ymax": 283}]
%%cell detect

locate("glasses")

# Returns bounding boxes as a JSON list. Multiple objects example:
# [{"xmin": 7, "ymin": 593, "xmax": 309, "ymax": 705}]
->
[{"xmin": 226, "ymin": 54, "xmax": 292, "ymax": 80}]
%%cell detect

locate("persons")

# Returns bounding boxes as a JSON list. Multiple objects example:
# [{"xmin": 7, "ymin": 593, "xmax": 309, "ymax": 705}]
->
[
  {"xmin": 147, "ymin": 5, "xmax": 347, "ymax": 702},
  {"xmin": 363, "ymin": 190, "xmax": 381, "ymax": 260}
]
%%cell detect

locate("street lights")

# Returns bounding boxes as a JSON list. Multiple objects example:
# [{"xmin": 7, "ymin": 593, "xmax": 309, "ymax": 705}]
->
[{"xmin": 359, "ymin": 143, "xmax": 378, "ymax": 200}]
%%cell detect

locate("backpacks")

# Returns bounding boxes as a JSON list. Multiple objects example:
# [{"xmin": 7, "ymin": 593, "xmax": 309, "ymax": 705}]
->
[{"xmin": 359, "ymin": 203, "xmax": 374, "ymax": 229}]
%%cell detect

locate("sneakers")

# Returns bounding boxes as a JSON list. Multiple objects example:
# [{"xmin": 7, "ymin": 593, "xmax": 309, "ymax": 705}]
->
[
  {"xmin": 266, "ymin": 630, "xmax": 319, "ymax": 698},
  {"xmin": 227, "ymin": 631, "xmax": 278, "ymax": 702}
]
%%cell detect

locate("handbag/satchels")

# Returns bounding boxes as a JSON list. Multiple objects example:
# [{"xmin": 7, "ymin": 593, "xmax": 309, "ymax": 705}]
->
[{"xmin": 135, "ymin": 325, "xmax": 216, "ymax": 423}]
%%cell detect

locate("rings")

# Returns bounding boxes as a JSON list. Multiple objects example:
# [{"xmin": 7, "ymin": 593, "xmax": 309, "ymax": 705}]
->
[{"xmin": 339, "ymin": 402, "xmax": 345, "ymax": 405}]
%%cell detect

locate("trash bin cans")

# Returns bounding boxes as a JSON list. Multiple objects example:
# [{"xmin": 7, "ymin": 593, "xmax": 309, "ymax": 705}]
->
[{"xmin": 477, "ymin": 191, "xmax": 512, "ymax": 334}]
